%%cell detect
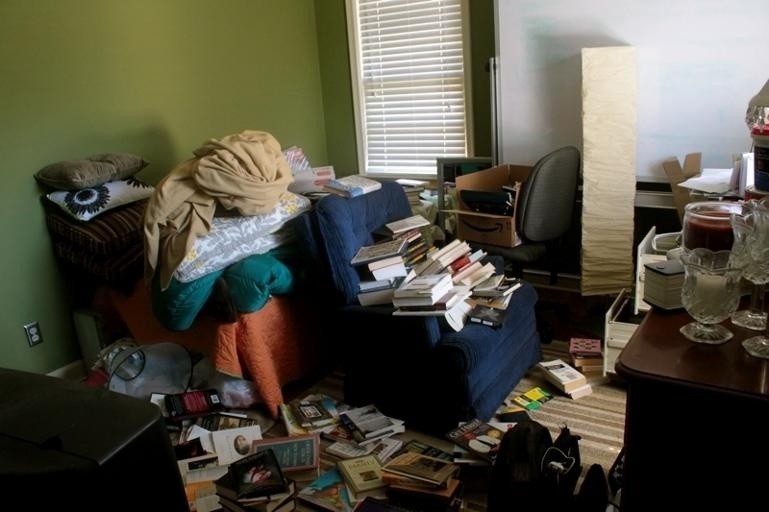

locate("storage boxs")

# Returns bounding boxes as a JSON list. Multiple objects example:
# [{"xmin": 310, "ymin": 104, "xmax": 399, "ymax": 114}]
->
[{"xmin": 438, "ymin": 162, "xmax": 535, "ymax": 248}]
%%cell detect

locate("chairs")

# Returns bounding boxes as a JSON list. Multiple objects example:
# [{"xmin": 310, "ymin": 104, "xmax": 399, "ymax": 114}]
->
[
  {"xmin": 433, "ymin": 145, "xmax": 581, "ymax": 285},
  {"xmin": 314, "ymin": 180, "xmax": 545, "ymax": 437}
]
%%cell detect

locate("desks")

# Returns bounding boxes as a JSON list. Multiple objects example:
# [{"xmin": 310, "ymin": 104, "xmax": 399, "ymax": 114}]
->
[{"xmin": 614, "ymin": 291, "xmax": 768, "ymax": 512}]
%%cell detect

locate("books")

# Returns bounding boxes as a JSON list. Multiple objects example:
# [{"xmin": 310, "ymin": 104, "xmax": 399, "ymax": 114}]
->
[
  {"xmin": 168, "ymin": 390, "xmax": 514, "ymax": 512},
  {"xmin": 326, "ymin": 173, "xmax": 381, "ymax": 200},
  {"xmin": 352, "ymin": 214, "xmax": 526, "ymax": 331},
  {"xmin": 511, "ymin": 338, "xmax": 605, "ymax": 412}
]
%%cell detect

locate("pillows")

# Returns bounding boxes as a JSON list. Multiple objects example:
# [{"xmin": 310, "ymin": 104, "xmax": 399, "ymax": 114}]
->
[
  {"xmin": 32, "ymin": 153, "xmax": 155, "ymax": 298},
  {"xmin": 172, "ymin": 224, "xmax": 308, "ymax": 283},
  {"xmin": 172, "ymin": 190, "xmax": 312, "ymax": 277}
]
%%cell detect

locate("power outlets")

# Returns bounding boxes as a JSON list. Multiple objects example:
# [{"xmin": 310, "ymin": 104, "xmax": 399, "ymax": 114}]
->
[{"xmin": 23, "ymin": 321, "xmax": 42, "ymax": 347}]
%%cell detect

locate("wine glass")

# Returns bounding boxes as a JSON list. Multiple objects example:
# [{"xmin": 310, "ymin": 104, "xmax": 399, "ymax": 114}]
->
[{"xmin": 678, "ymin": 198, "xmax": 769, "ymax": 363}]
